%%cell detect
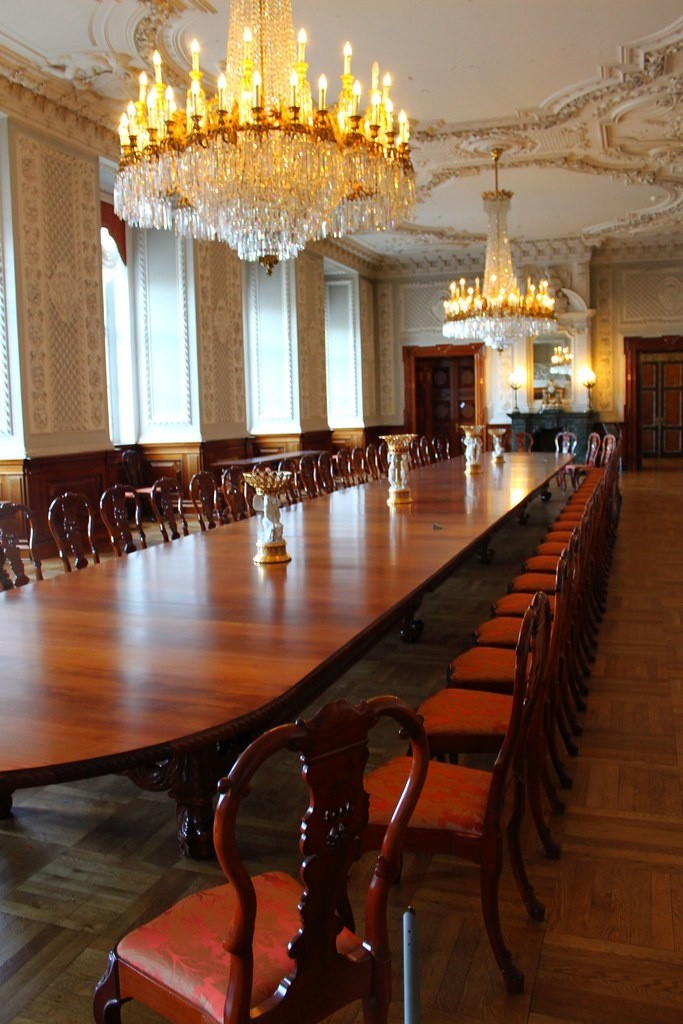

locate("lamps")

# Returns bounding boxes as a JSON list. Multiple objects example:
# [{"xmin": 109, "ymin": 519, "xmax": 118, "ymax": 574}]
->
[
  {"xmin": 507, "ymin": 369, "xmax": 523, "ymax": 412},
  {"xmin": 580, "ymin": 367, "xmax": 596, "ymax": 412},
  {"xmin": 442, "ymin": 149, "xmax": 557, "ymax": 357},
  {"xmin": 550, "ymin": 335, "xmax": 572, "ymax": 375},
  {"xmin": 114, "ymin": 0, "xmax": 415, "ymax": 274}
]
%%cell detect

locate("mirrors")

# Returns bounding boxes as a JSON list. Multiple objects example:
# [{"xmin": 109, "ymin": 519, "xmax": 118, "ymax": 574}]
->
[{"xmin": 526, "ymin": 327, "xmax": 580, "ymax": 413}]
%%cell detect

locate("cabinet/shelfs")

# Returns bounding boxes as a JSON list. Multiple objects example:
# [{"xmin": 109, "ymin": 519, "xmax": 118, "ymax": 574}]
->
[{"xmin": 507, "ymin": 411, "xmax": 598, "ymax": 463}]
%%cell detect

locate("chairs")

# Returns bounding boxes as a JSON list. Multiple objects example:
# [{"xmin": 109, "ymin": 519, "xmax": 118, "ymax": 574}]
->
[{"xmin": 0, "ymin": 431, "xmax": 620, "ymax": 1024}]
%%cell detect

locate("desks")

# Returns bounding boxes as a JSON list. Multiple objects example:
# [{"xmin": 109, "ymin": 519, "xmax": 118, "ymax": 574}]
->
[{"xmin": 0, "ymin": 453, "xmax": 576, "ymax": 864}]
